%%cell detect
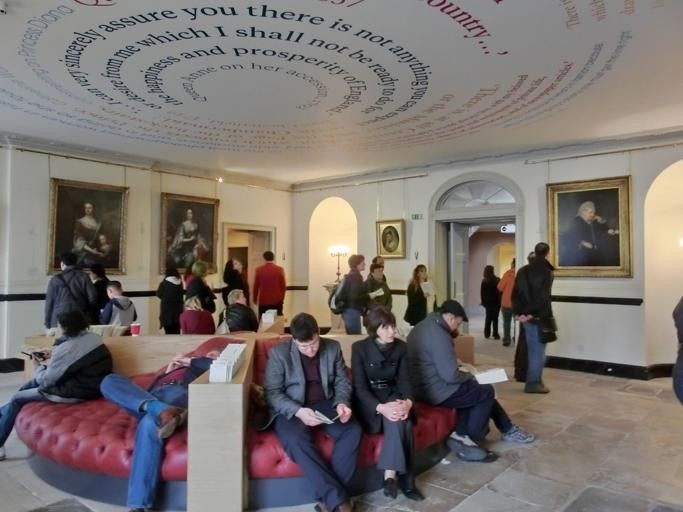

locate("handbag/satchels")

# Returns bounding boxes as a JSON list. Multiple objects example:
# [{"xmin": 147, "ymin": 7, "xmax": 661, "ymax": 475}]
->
[
  {"xmin": 538, "ymin": 319, "xmax": 558, "ymax": 343},
  {"xmin": 214, "ymin": 318, "xmax": 230, "ymax": 336}
]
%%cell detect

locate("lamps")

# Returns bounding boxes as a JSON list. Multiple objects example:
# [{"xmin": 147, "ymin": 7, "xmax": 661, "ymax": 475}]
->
[{"xmin": 327, "ymin": 244, "xmax": 351, "ymax": 285}]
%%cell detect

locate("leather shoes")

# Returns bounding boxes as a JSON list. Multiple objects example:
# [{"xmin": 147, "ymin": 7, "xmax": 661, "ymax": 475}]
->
[
  {"xmin": 399, "ymin": 488, "xmax": 425, "ymax": 501},
  {"xmin": 447, "ymin": 437, "xmax": 498, "ymax": 462},
  {"xmin": 158, "ymin": 406, "xmax": 187, "ymax": 439},
  {"xmin": 314, "ymin": 499, "xmax": 354, "ymax": 512},
  {"xmin": 383, "ymin": 478, "xmax": 397, "ymax": 497}
]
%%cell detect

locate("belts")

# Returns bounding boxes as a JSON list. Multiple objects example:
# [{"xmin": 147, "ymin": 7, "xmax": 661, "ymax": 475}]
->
[
  {"xmin": 163, "ymin": 380, "xmax": 183, "ymax": 386},
  {"xmin": 372, "ymin": 384, "xmax": 387, "ymax": 388}
]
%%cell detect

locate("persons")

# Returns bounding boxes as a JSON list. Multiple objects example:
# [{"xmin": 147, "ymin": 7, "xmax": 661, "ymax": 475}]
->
[
  {"xmin": 514, "ymin": 242, "xmax": 558, "ymax": 393},
  {"xmin": 403, "ymin": 264, "xmax": 438, "ymax": 327},
  {"xmin": 339, "ymin": 254, "xmax": 392, "ymax": 335},
  {"xmin": 349, "ymin": 306, "xmax": 425, "ymax": 501},
  {"xmin": 0, "ymin": 310, "xmax": 112, "ymax": 461},
  {"xmin": 252, "ymin": 313, "xmax": 363, "ymax": 512},
  {"xmin": 383, "ymin": 227, "xmax": 400, "ymax": 252},
  {"xmin": 164, "ymin": 206, "xmax": 215, "ymax": 276},
  {"xmin": 99, "ymin": 348, "xmax": 222, "ymax": 511},
  {"xmin": 407, "ymin": 299, "xmax": 536, "ymax": 463},
  {"xmin": 252, "ymin": 251, "xmax": 286, "ymax": 321},
  {"xmin": 480, "ymin": 252, "xmax": 543, "ymax": 382},
  {"xmin": 44, "ymin": 251, "xmax": 138, "ymax": 337},
  {"xmin": 156, "ymin": 257, "xmax": 259, "ymax": 334},
  {"xmin": 559, "ymin": 200, "xmax": 617, "ymax": 266},
  {"xmin": 72, "ymin": 201, "xmax": 117, "ymax": 267}
]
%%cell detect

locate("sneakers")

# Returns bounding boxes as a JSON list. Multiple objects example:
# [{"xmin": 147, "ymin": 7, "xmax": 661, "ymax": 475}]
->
[
  {"xmin": 495, "ymin": 335, "xmax": 500, "ymax": 339},
  {"xmin": 524, "ymin": 382, "xmax": 550, "ymax": 393},
  {"xmin": 502, "ymin": 424, "xmax": 535, "ymax": 443}
]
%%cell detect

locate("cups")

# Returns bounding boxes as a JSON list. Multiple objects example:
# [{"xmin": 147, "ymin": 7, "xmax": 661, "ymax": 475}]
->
[{"xmin": 130, "ymin": 324, "xmax": 140, "ymax": 336}]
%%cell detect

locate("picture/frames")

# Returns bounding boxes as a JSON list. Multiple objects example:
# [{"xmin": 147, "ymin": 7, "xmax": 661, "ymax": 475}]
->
[
  {"xmin": 375, "ymin": 220, "xmax": 407, "ymax": 259},
  {"xmin": 45, "ymin": 176, "xmax": 130, "ymax": 277},
  {"xmin": 158, "ymin": 191, "xmax": 220, "ymax": 276},
  {"xmin": 545, "ymin": 174, "xmax": 633, "ymax": 279}
]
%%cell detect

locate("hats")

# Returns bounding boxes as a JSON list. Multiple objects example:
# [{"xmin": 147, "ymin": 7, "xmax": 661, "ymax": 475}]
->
[{"xmin": 442, "ymin": 300, "xmax": 468, "ymax": 323}]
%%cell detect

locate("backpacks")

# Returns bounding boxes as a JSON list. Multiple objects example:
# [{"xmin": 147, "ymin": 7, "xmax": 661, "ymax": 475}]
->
[{"xmin": 329, "ymin": 272, "xmax": 360, "ymax": 315}]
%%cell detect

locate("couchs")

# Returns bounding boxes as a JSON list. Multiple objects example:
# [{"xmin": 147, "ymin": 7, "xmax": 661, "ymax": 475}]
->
[{"xmin": 14, "ymin": 331, "xmax": 477, "ymax": 511}]
